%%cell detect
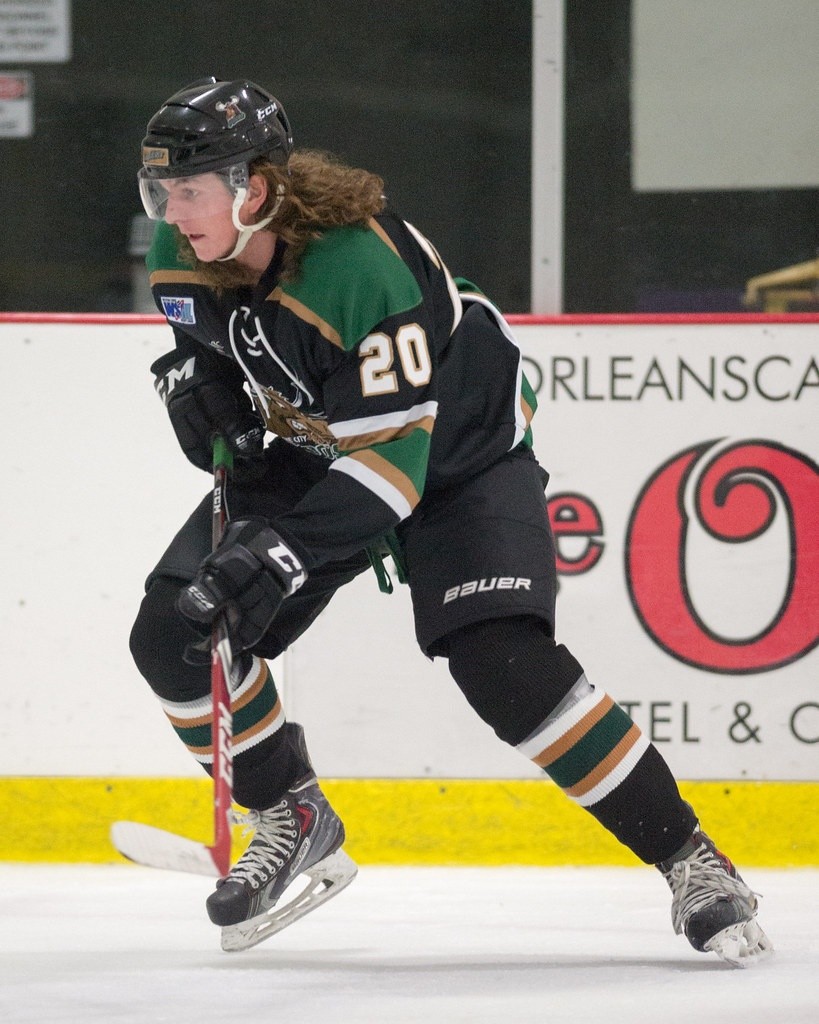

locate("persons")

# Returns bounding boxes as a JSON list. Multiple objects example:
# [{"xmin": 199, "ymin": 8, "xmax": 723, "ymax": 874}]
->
[{"xmin": 129, "ymin": 77, "xmax": 776, "ymax": 969}]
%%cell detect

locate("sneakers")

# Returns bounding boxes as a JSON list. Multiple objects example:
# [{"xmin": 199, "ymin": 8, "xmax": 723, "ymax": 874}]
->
[
  {"xmin": 206, "ymin": 722, "xmax": 358, "ymax": 952},
  {"xmin": 655, "ymin": 831, "xmax": 774, "ymax": 968}
]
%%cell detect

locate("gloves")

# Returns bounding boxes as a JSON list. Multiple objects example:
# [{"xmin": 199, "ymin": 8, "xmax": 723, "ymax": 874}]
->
[
  {"xmin": 150, "ymin": 340, "xmax": 266, "ymax": 475},
  {"xmin": 174, "ymin": 516, "xmax": 309, "ymax": 666}
]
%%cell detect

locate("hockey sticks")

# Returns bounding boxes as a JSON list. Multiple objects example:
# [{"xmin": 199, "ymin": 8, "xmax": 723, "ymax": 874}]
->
[{"xmin": 107, "ymin": 423, "xmax": 237, "ymax": 881}]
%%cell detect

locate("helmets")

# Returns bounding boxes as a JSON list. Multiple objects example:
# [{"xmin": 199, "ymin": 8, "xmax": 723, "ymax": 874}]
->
[{"xmin": 138, "ymin": 77, "xmax": 294, "ymax": 181}]
